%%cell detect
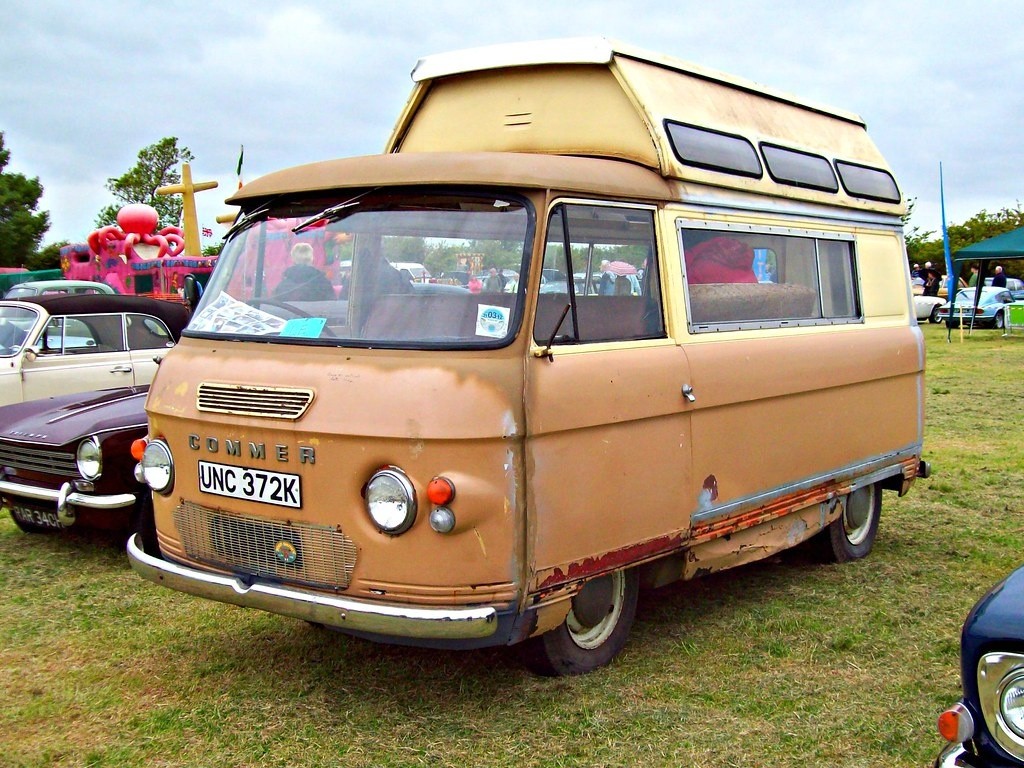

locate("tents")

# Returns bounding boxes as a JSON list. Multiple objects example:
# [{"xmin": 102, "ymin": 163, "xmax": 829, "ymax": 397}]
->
[{"xmin": 946, "ymin": 226, "xmax": 1024, "ymax": 343}]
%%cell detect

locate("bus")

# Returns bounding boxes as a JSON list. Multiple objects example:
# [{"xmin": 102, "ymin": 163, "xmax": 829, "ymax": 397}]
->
[{"xmin": 126, "ymin": 36, "xmax": 929, "ymax": 677}]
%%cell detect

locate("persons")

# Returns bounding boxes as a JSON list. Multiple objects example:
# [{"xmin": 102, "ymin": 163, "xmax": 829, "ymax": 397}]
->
[
  {"xmin": 910, "ymin": 261, "xmax": 940, "ymax": 297},
  {"xmin": 338, "ymin": 236, "xmax": 413, "ymax": 302},
  {"xmin": 484, "ymin": 268, "xmax": 519, "ymax": 294},
  {"xmin": 991, "ymin": 265, "xmax": 1006, "ymax": 288},
  {"xmin": 598, "ymin": 260, "xmax": 615, "ymax": 295},
  {"xmin": 626, "ymin": 274, "xmax": 643, "ymax": 296},
  {"xmin": 969, "ymin": 264, "xmax": 979, "ymax": 288},
  {"xmin": 467, "ymin": 275, "xmax": 483, "ymax": 294},
  {"xmin": 682, "ymin": 229, "xmax": 760, "ymax": 285},
  {"xmin": 615, "ymin": 275, "xmax": 632, "ymax": 296},
  {"xmin": 272, "ymin": 241, "xmax": 338, "ymax": 302}
]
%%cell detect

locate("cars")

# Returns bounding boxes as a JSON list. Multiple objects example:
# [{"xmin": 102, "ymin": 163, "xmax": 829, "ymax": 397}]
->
[
  {"xmin": 0, "ymin": 288, "xmax": 176, "ymax": 405},
  {"xmin": 911, "ymin": 276, "xmax": 969, "ymax": 297},
  {"xmin": 932, "ymin": 562, "xmax": 1024, "ymax": 768},
  {"xmin": 540, "ymin": 267, "xmax": 602, "ymax": 287},
  {"xmin": 440, "ymin": 271, "xmax": 470, "ymax": 285},
  {"xmin": 1010, "ymin": 290, "xmax": 1024, "ymax": 300},
  {"xmin": 340, "ymin": 261, "xmax": 352, "ymax": 281},
  {"xmin": 937, "ymin": 286, "xmax": 1015, "ymax": 328},
  {"xmin": 0, "ymin": 386, "xmax": 152, "ymax": 542},
  {"xmin": 913, "ymin": 294, "xmax": 947, "ymax": 324},
  {"xmin": 476, "ymin": 267, "xmax": 519, "ymax": 284},
  {"xmin": 5, "ymin": 279, "xmax": 119, "ymax": 298}
]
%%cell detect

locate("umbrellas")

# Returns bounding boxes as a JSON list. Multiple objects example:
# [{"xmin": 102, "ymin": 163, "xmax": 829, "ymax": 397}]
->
[
  {"xmin": 610, "ymin": 261, "xmax": 639, "ymax": 275},
  {"xmin": 917, "ymin": 267, "xmax": 942, "ymax": 282}
]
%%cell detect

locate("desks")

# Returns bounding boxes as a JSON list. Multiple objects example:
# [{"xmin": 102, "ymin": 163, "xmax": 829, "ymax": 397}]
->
[{"xmin": 1002, "ymin": 302, "xmax": 1024, "ymax": 337}]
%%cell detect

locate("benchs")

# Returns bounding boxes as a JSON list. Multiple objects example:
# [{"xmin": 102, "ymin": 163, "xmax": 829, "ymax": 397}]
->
[{"xmin": 359, "ymin": 283, "xmax": 818, "ymax": 338}]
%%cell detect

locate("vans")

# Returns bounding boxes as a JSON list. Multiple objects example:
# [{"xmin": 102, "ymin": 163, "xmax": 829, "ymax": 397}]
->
[
  {"xmin": 983, "ymin": 276, "xmax": 1024, "ymax": 290},
  {"xmin": 390, "ymin": 262, "xmax": 433, "ymax": 284}
]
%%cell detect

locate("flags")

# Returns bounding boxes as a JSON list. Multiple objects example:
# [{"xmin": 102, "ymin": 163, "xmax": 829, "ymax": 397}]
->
[{"xmin": 236, "ymin": 144, "xmax": 244, "ymax": 189}]
